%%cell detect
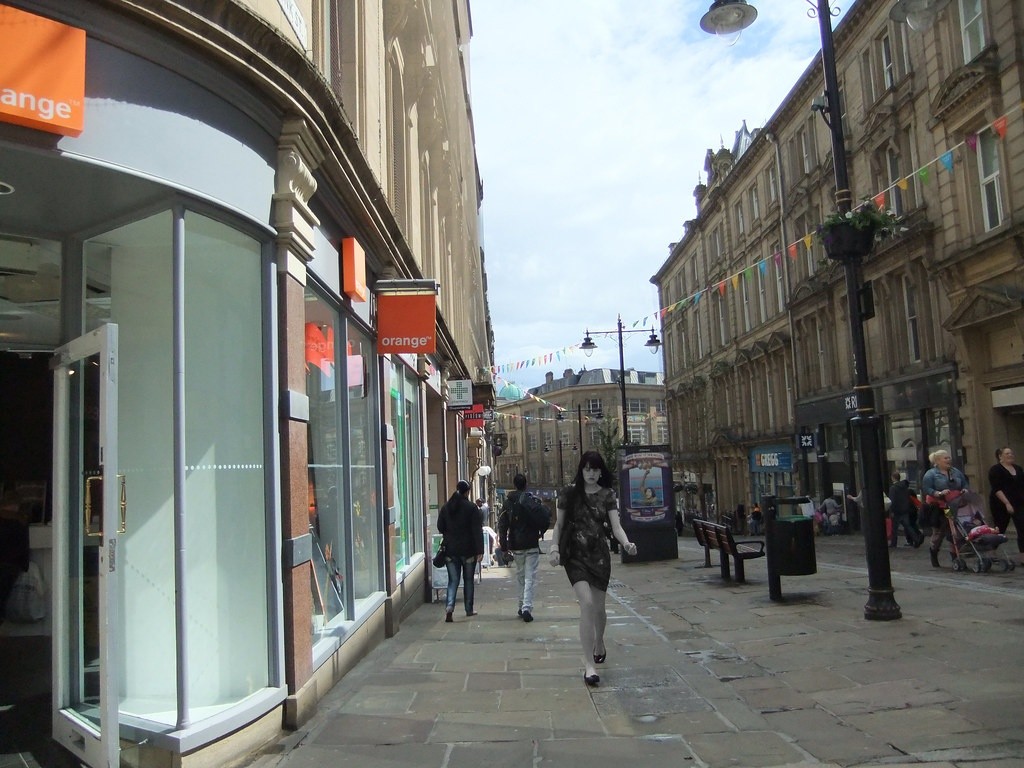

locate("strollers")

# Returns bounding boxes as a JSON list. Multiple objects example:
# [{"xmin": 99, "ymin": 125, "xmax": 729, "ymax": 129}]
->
[{"xmin": 938, "ymin": 489, "xmax": 1016, "ymax": 574}]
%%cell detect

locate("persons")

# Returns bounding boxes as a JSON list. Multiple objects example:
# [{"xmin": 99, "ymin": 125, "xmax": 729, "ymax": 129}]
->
[
  {"xmin": 476, "ymin": 497, "xmax": 488, "ymax": 526},
  {"xmin": 437, "ymin": 479, "xmax": 484, "ymax": 621},
  {"xmin": 640, "ymin": 469, "xmax": 658, "ymax": 504},
  {"xmin": 815, "ymin": 492, "xmax": 843, "ymax": 536},
  {"xmin": 498, "ymin": 474, "xmax": 550, "ymax": 622},
  {"xmin": 922, "ymin": 450, "xmax": 969, "ymax": 567},
  {"xmin": 550, "ymin": 545, "xmax": 559, "ymax": 554},
  {"xmin": 550, "ymin": 451, "xmax": 637, "ymax": 681},
  {"xmin": 750, "ymin": 502, "xmax": 761, "ymax": 536},
  {"xmin": 847, "ymin": 490, "xmax": 864, "ymax": 535},
  {"xmin": 988, "ymin": 447, "xmax": 1024, "ymax": 562},
  {"xmin": 888, "ymin": 472, "xmax": 925, "ymax": 547},
  {"xmin": 0, "ymin": 463, "xmax": 32, "ymax": 599},
  {"xmin": 676, "ymin": 511, "xmax": 683, "ymax": 537},
  {"xmin": 965, "ymin": 522, "xmax": 999, "ymax": 534}
]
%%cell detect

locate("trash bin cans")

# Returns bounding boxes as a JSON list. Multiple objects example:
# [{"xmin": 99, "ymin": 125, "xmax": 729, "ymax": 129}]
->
[{"xmin": 773, "ymin": 496, "xmax": 817, "ymax": 576}]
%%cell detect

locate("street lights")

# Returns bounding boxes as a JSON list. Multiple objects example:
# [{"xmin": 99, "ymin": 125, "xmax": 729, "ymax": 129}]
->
[
  {"xmin": 544, "ymin": 440, "xmax": 579, "ymax": 486},
  {"xmin": 698, "ymin": 0, "xmax": 903, "ymax": 624},
  {"xmin": 578, "ymin": 311, "xmax": 662, "ymax": 445},
  {"xmin": 554, "ymin": 403, "xmax": 604, "ymax": 456}
]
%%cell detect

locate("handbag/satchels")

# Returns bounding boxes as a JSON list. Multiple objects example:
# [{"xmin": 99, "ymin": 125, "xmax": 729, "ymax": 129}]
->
[
  {"xmin": 433, "ymin": 547, "xmax": 446, "ymax": 568},
  {"xmin": 918, "ymin": 502, "xmax": 945, "ymax": 528},
  {"xmin": 885, "ymin": 517, "xmax": 893, "ymax": 539},
  {"xmin": 517, "ymin": 492, "xmax": 552, "ymax": 532}
]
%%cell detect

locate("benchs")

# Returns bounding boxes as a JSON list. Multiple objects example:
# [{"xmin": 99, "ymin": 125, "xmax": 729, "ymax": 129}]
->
[{"xmin": 692, "ymin": 519, "xmax": 765, "ymax": 582}]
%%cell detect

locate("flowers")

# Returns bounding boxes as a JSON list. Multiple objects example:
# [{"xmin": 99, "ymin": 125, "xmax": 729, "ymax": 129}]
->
[{"xmin": 817, "ymin": 194, "xmax": 908, "ymax": 267}]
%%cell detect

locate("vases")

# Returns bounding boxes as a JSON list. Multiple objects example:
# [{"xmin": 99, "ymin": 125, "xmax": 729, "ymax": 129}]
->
[{"xmin": 824, "ymin": 224, "xmax": 875, "ymax": 259}]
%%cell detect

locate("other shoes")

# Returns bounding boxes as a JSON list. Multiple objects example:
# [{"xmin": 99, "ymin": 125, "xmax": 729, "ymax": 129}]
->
[
  {"xmin": 929, "ymin": 547, "xmax": 941, "ymax": 567},
  {"xmin": 1021, "ymin": 562, "xmax": 1024, "ymax": 566},
  {"xmin": 919, "ymin": 534, "xmax": 925, "ymax": 545},
  {"xmin": 472, "ymin": 610, "xmax": 477, "ymax": 615},
  {"xmin": 522, "ymin": 610, "xmax": 533, "ymax": 622},
  {"xmin": 518, "ymin": 608, "xmax": 522, "ymax": 616},
  {"xmin": 446, "ymin": 608, "xmax": 453, "ymax": 622},
  {"xmin": 584, "ymin": 671, "xmax": 601, "ymax": 683},
  {"xmin": 904, "ymin": 543, "xmax": 913, "ymax": 546},
  {"xmin": 593, "ymin": 640, "xmax": 606, "ymax": 663},
  {"xmin": 991, "ymin": 558, "xmax": 999, "ymax": 564},
  {"xmin": 915, "ymin": 536, "xmax": 920, "ymax": 548},
  {"xmin": 888, "ymin": 544, "xmax": 897, "ymax": 548},
  {"xmin": 950, "ymin": 552, "xmax": 957, "ymax": 562}
]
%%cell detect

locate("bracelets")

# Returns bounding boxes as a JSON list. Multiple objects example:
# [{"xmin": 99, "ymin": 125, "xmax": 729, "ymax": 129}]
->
[
  {"xmin": 939, "ymin": 491, "xmax": 942, "ymax": 497},
  {"xmin": 623, "ymin": 541, "xmax": 629, "ymax": 546}
]
